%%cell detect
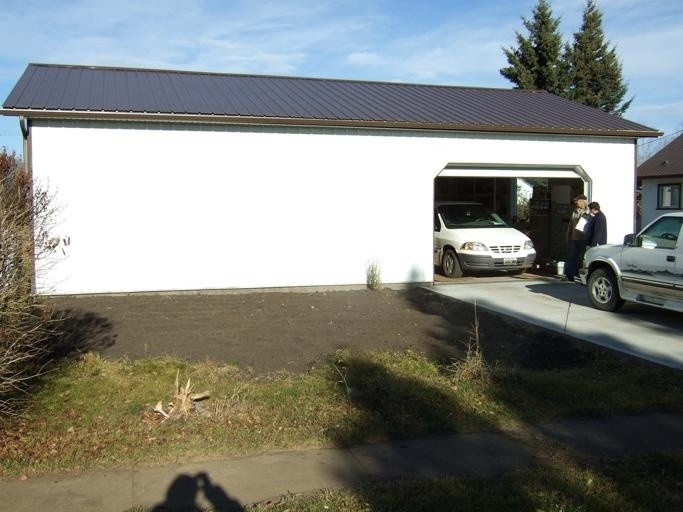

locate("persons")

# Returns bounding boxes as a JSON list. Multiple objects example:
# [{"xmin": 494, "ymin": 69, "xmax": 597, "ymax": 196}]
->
[
  {"xmin": 581, "ymin": 201, "xmax": 607, "ymax": 246},
  {"xmin": 561, "ymin": 193, "xmax": 595, "ymax": 281}
]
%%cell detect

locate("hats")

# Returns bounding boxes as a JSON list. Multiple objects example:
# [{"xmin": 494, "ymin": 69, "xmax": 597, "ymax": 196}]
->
[{"xmin": 573, "ymin": 194, "xmax": 587, "ymax": 201}]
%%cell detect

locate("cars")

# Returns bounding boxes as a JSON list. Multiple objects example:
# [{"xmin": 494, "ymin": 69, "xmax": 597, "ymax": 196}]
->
[
  {"xmin": 434, "ymin": 200, "xmax": 537, "ymax": 278},
  {"xmin": 578, "ymin": 211, "xmax": 683, "ymax": 313}
]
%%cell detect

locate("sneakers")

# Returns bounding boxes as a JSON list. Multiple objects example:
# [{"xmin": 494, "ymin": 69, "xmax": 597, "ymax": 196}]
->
[{"xmin": 561, "ymin": 276, "xmax": 576, "ymax": 281}]
